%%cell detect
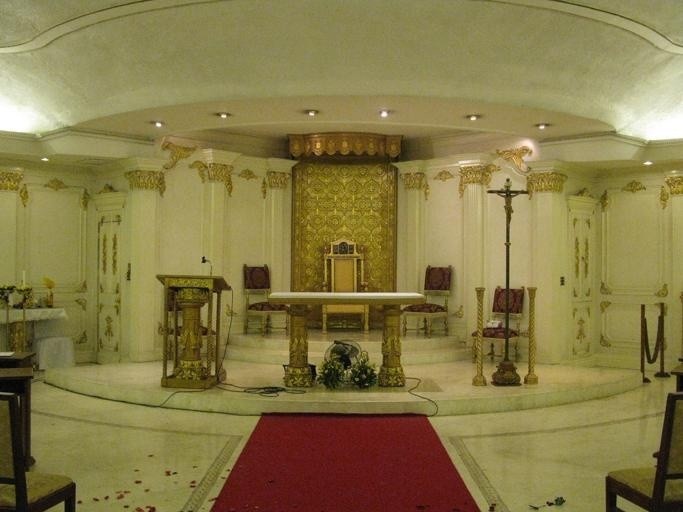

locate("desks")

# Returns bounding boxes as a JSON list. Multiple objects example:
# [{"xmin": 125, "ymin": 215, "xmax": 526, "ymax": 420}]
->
[
  {"xmin": 269, "ymin": 292, "xmax": 426, "ymax": 388},
  {"xmin": 0, "ymin": 307, "xmax": 68, "ymax": 352},
  {"xmin": 0, "ymin": 351, "xmax": 37, "ymax": 472},
  {"xmin": 670, "ymin": 362, "xmax": 683, "ymax": 394}
]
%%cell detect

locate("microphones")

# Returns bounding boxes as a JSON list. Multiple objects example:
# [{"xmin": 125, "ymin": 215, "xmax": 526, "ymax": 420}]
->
[{"xmin": 201, "ymin": 256, "xmax": 213, "ymax": 277}]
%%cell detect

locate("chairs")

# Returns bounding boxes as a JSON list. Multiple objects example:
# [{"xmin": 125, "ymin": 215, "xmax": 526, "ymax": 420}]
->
[
  {"xmin": 604, "ymin": 392, "xmax": 683, "ymax": 512},
  {"xmin": 402, "ymin": 265, "xmax": 452, "ymax": 338},
  {"xmin": 0, "ymin": 391, "xmax": 76, "ymax": 512},
  {"xmin": 320, "ymin": 237, "xmax": 371, "ymax": 331},
  {"xmin": 471, "ymin": 285, "xmax": 525, "ymax": 362},
  {"xmin": 243, "ymin": 264, "xmax": 290, "ymax": 337}
]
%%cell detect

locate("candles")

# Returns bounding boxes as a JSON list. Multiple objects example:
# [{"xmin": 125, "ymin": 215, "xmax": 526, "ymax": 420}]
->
[{"xmin": 22, "ymin": 270, "xmax": 26, "ymax": 290}]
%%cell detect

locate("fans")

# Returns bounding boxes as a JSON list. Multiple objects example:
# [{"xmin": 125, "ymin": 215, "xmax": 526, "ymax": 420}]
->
[{"xmin": 323, "ymin": 339, "xmax": 362, "ymax": 370}]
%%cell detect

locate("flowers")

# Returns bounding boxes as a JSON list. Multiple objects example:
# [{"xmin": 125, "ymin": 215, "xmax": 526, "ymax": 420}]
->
[
  {"xmin": 315, "ymin": 358, "xmax": 346, "ymax": 389},
  {"xmin": 349, "ymin": 362, "xmax": 377, "ymax": 386}
]
